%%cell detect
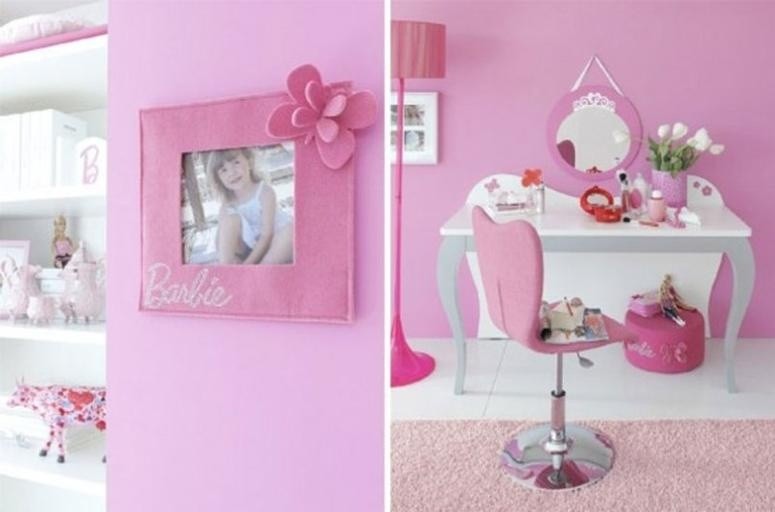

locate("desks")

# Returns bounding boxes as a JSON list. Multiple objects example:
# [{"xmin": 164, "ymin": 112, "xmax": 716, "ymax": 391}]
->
[{"xmin": 423, "ymin": 170, "xmax": 757, "ymax": 396}]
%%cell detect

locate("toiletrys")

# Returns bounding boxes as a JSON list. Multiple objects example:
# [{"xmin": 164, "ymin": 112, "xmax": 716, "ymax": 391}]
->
[{"xmin": 622, "ymin": 173, "xmax": 666, "ymax": 221}]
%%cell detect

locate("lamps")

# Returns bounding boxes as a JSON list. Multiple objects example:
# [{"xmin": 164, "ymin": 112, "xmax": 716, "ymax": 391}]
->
[{"xmin": 386, "ymin": 18, "xmax": 447, "ymax": 389}]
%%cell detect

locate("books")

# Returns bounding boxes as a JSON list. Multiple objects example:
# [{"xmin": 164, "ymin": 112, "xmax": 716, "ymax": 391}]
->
[{"xmin": 540, "ymin": 301, "xmax": 609, "ymax": 344}]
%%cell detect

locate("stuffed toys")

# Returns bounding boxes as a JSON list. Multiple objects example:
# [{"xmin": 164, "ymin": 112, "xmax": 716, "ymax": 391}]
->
[{"xmin": 51, "ymin": 216, "xmax": 73, "ymax": 268}]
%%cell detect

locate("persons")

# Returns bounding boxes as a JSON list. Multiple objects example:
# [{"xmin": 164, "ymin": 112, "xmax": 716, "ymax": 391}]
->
[
  {"xmin": 406, "ymin": 131, "xmax": 419, "ymax": 151},
  {"xmin": 208, "ymin": 148, "xmax": 294, "ymax": 265},
  {"xmin": 407, "ymin": 105, "xmax": 424, "ymax": 125}
]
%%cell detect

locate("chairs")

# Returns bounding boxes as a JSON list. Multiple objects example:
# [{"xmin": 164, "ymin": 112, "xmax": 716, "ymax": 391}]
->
[{"xmin": 470, "ymin": 203, "xmax": 632, "ymax": 492}]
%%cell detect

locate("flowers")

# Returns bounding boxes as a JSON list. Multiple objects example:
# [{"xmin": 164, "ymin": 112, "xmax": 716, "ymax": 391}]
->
[{"xmin": 647, "ymin": 122, "xmax": 727, "ymax": 176}]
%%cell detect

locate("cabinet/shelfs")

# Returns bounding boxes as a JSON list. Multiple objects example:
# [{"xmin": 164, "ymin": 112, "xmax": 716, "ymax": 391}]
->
[{"xmin": 0, "ymin": 26, "xmax": 108, "ymax": 498}]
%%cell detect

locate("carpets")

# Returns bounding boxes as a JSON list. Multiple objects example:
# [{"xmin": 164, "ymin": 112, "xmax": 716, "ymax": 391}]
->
[{"xmin": 390, "ymin": 413, "xmax": 775, "ymax": 512}]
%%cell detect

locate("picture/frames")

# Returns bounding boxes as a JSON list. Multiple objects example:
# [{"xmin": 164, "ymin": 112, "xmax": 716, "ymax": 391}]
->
[
  {"xmin": 137, "ymin": 78, "xmax": 358, "ymax": 323},
  {"xmin": 388, "ymin": 91, "xmax": 442, "ymax": 165}
]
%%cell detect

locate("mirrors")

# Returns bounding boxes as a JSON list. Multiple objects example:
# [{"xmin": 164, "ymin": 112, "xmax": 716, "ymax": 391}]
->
[{"xmin": 544, "ymin": 90, "xmax": 645, "ymax": 183}]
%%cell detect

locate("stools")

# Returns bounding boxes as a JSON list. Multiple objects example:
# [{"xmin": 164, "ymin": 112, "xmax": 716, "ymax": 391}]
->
[{"xmin": 624, "ymin": 306, "xmax": 704, "ymax": 373}]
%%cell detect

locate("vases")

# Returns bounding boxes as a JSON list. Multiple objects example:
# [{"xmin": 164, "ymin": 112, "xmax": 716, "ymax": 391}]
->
[{"xmin": 652, "ymin": 168, "xmax": 690, "ymax": 206}]
